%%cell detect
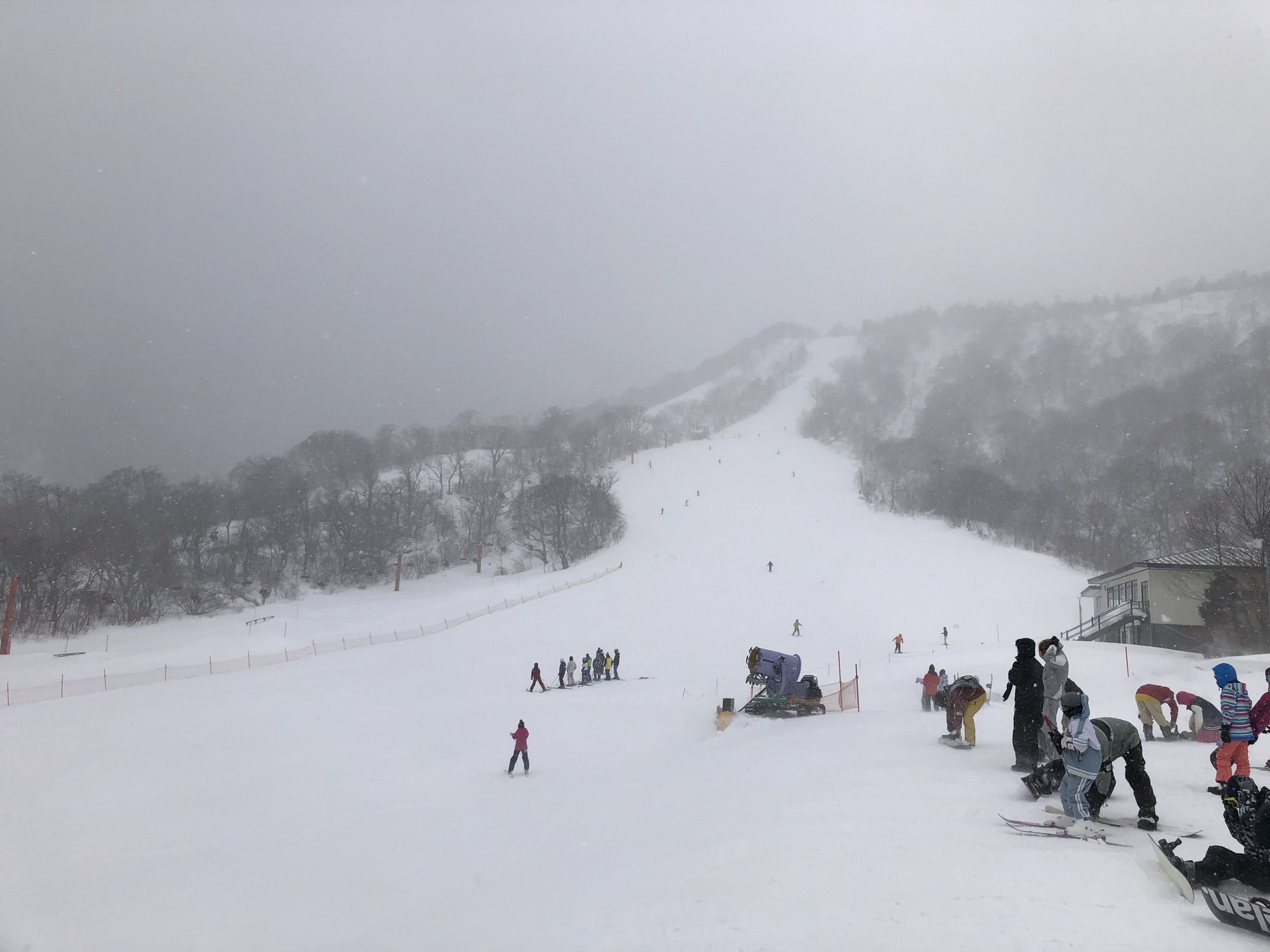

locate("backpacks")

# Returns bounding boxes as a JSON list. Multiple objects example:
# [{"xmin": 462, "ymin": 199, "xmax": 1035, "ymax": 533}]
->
[{"xmin": 949, "ymin": 675, "xmax": 978, "ymax": 703}]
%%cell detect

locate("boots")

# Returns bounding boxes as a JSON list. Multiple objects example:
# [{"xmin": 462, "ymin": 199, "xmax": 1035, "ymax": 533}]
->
[
  {"xmin": 1159, "ymin": 725, "xmax": 1177, "ymax": 741},
  {"xmin": 1143, "ymin": 723, "xmax": 1156, "ymax": 742}
]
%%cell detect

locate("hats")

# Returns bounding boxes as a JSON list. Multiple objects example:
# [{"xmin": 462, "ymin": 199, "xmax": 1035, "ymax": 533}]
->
[
  {"xmin": 1212, "ymin": 663, "xmax": 1237, "ymax": 688},
  {"xmin": 1060, "ymin": 692, "xmax": 1082, "ymax": 707},
  {"xmin": 929, "ymin": 664, "xmax": 947, "ymax": 707},
  {"xmin": 1227, "ymin": 775, "xmax": 1260, "ymax": 798},
  {"xmin": 518, "ymin": 719, "xmax": 524, "ymax": 727}
]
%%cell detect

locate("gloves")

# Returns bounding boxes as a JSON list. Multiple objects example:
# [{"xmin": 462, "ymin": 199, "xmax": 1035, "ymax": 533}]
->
[
  {"xmin": 1048, "ymin": 731, "xmax": 1063, "ymax": 755},
  {"xmin": 1236, "ymin": 775, "xmax": 1257, "ymax": 793},
  {"xmin": 1219, "ymin": 723, "xmax": 1231, "ymax": 743},
  {"xmin": 1002, "ymin": 682, "xmax": 1013, "ymax": 701},
  {"xmin": 947, "ymin": 723, "xmax": 954, "ymax": 732},
  {"xmin": 1008, "ymin": 661, "xmax": 1020, "ymax": 676},
  {"xmin": 1223, "ymin": 797, "xmax": 1237, "ymax": 814}
]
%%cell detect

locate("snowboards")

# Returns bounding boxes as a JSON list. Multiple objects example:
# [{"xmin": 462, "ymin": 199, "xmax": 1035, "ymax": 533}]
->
[
  {"xmin": 938, "ymin": 735, "xmax": 972, "ymax": 748},
  {"xmin": 1145, "ymin": 832, "xmax": 1195, "ymax": 904},
  {"xmin": 1020, "ymin": 772, "xmax": 1041, "ymax": 802},
  {"xmin": 1042, "ymin": 803, "xmax": 1208, "ymax": 838},
  {"xmin": 1199, "ymin": 885, "xmax": 1270, "ymax": 939},
  {"xmin": 1210, "ymin": 692, "xmax": 1270, "ymax": 771}
]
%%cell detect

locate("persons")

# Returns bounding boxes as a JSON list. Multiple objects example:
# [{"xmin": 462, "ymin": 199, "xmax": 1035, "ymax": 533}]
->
[
  {"xmin": 1008, "ymin": 636, "xmax": 1159, "ymax": 830},
  {"xmin": 892, "ymin": 633, "xmax": 903, "ymax": 653},
  {"xmin": 776, "ymin": 450, "xmax": 780, "ymax": 454},
  {"xmin": 697, "ymin": 491, "xmax": 700, "ymax": 496},
  {"xmin": 792, "ymin": 619, "xmax": 802, "ymax": 636},
  {"xmin": 941, "ymin": 627, "xmax": 948, "ymax": 644},
  {"xmin": 792, "ymin": 471, "xmax": 794, "ymax": 476},
  {"xmin": 567, "ymin": 647, "xmax": 620, "ymax": 686},
  {"xmin": 917, "ymin": 664, "xmax": 949, "ymax": 712},
  {"xmin": 507, "ymin": 719, "xmax": 530, "ymax": 774},
  {"xmin": 1048, "ymin": 692, "xmax": 1102, "ymax": 838},
  {"xmin": 529, "ymin": 663, "xmax": 547, "ymax": 693},
  {"xmin": 767, "ymin": 561, "xmax": 774, "ymax": 571},
  {"xmin": 718, "ymin": 459, "xmax": 721, "ymax": 464},
  {"xmin": 661, "ymin": 507, "xmax": 664, "ymax": 514},
  {"xmin": 709, "ymin": 445, "xmax": 711, "ymax": 450},
  {"xmin": 649, "ymin": 461, "xmax": 651, "ymax": 467},
  {"xmin": 1135, "ymin": 663, "xmax": 1270, "ymax": 892},
  {"xmin": 558, "ymin": 658, "xmax": 566, "ymax": 688},
  {"xmin": 685, "ymin": 499, "xmax": 689, "ymax": 506},
  {"xmin": 934, "ymin": 675, "xmax": 987, "ymax": 747}
]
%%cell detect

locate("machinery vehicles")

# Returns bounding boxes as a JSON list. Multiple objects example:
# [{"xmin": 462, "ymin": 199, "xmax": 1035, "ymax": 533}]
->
[{"xmin": 737, "ymin": 646, "xmax": 825, "ymax": 716}]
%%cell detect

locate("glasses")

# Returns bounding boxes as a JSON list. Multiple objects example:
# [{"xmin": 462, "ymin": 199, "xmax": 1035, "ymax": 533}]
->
[
  {"xmin": 1214, "ymin": 675, "xmax": 1217, "ymax": 680},
  {"xmin": 1224, "ymin": 796, "xmax": 1239, "ymax": 808}
]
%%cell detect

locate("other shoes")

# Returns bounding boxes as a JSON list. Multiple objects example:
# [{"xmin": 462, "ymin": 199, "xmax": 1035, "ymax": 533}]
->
[
  {"xmin": 1158, "ymin": 838, "xmax": 1195, "ymax": 883},
  {"xmin": 942, "ymin": 733, "xmax": 962, "ymax": 740},
  {"xmin": 1011, "ymin": 762, "xmax": 1037, "ymax": 773},
  {"xmin": 529, "ymin": 673, "xmax": 620, "ymax": 692},
  {"xmin": 1044, "ymin": 796, "xmax": 1159, "ymax": 838},
  {"xmin": 1207, "ymin": 781, "xmax": 1227, "ymax": 795},
  {"xmin": 1181, "ymin": 730, "xmax": 1197, "ymax": 741}
]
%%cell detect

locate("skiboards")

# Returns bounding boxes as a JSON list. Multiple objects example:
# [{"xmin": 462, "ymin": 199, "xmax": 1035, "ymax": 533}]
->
[
  {"xmin": 557, "ymin": 676, "xmax": 625, "ymax": 687},
  {"xmin": 525, "ymin": 688, "xmax": 552, "ymax": 693},
  {"xmin": 995, "ymin": 811, "xmax": 1133, "ymax": 846},
  {"xmin": 551, "ymin": 686, "xmax": 573, "ymax": 690},
  {"xmin": 504, "ymin": 769, "xmax": 530, "ymax": 778}
]
